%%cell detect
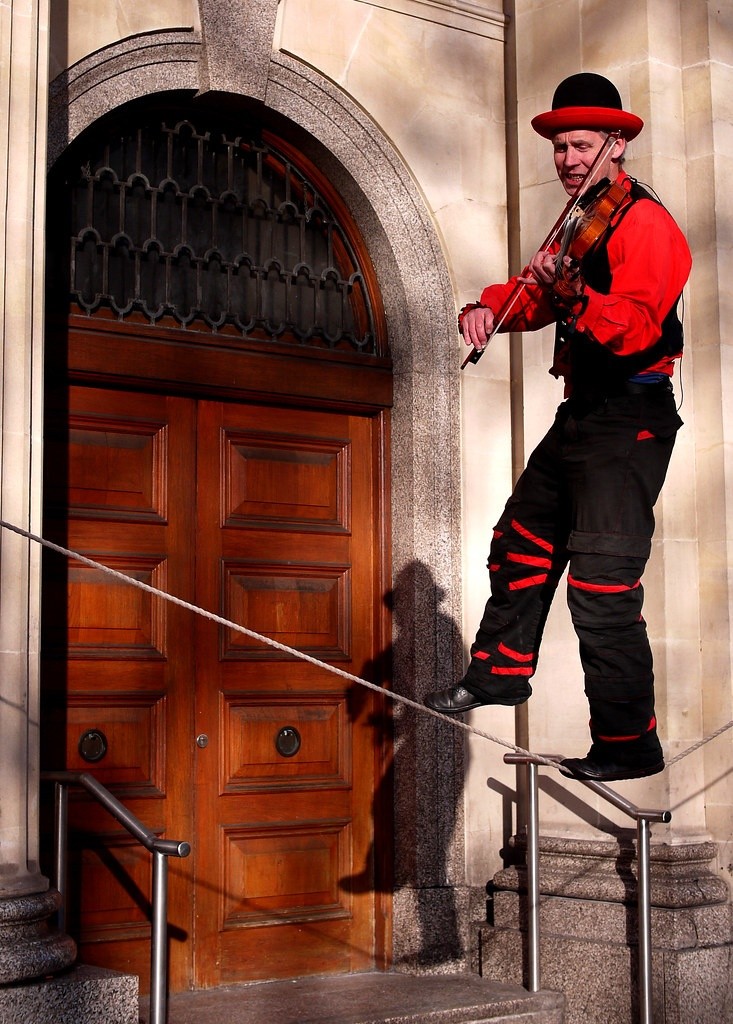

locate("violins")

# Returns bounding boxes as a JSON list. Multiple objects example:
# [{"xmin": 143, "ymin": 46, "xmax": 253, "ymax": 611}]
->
[{"xmin": 552, "ymin": 180, "xmax": 627, "ymax": 298}]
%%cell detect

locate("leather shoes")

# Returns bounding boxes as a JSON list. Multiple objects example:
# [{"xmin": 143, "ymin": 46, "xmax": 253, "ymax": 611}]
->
[
  {"xmin": 558, "ymin": 732, "xmax": 665, "ymax": 781},
  {"xmin": 423, "ymin": 684, "xmax": 532, "ymax": 713}
]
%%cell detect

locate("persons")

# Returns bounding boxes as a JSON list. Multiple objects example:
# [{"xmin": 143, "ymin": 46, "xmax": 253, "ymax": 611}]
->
[{"xmin": 423, "ymin": 72, "xmax": 692, "ymax": 782}]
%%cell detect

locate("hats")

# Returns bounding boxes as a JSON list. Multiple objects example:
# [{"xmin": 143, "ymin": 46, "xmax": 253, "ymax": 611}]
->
[{"xmin": 531, "ymin": 72, "xmax": 643, "ymax": 143}]
